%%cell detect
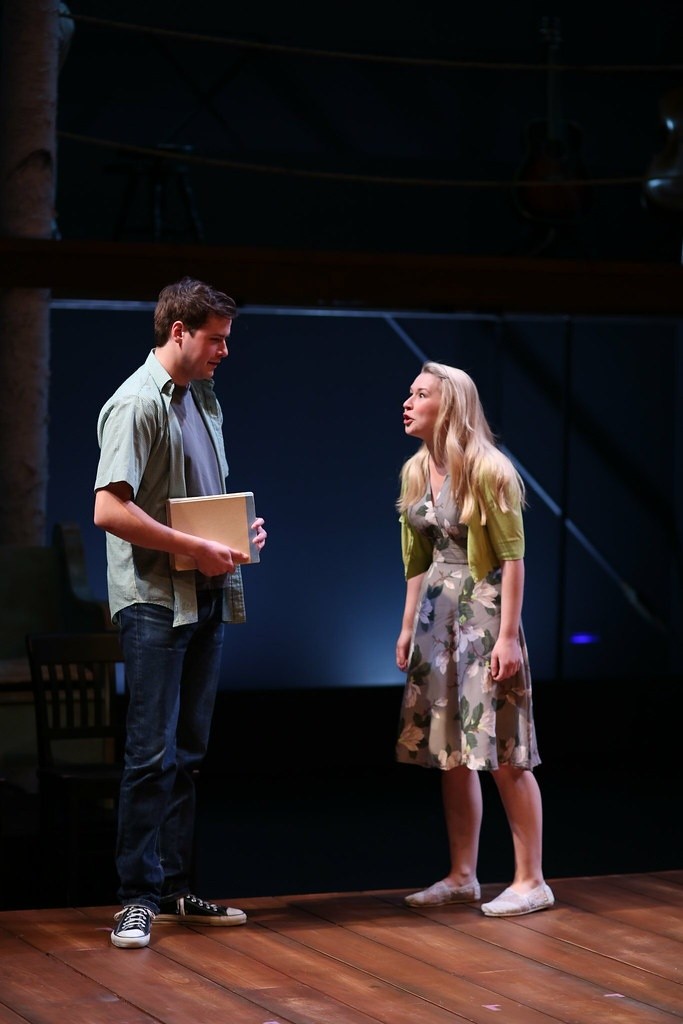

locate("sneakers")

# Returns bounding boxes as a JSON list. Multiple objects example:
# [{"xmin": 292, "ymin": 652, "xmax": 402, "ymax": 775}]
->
[
  {"xmin": 111, "ymin": 906, "xmax": 156, "ymax": 948},
  {"xmin": 151, "ymin": 894, "xmax": 246, "ymax": 926}
]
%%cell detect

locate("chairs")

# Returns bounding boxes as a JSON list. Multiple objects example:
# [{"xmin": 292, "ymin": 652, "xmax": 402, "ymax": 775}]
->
[
  {"xmin": 27, "ymin": 632, "xmax": 196, "ymax": 896},
  {"xmin": 0, "ymin": 521, "xmax": 126, "ymax": 812}
]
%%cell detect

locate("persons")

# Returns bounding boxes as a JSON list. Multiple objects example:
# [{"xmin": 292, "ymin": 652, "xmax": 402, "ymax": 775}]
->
[
  {"xmin": 395, "ymin": 362, "xmax": 555, "ymax": 917},
  {"xmin": 94, "ymin": 278, "xmax": 268, "ymax": 949}
]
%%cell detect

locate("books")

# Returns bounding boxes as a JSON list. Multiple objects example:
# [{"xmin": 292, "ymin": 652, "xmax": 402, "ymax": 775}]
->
[{"xmin": 166, "ymin": 492, "xmax": 260, "ymax": 571}]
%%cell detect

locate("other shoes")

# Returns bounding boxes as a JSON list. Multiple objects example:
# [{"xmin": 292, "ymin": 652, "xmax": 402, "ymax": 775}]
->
[
  {"xmin": 405, "ymin": 881, "xmax": 481, "ymax": 906},
  {"xmin": 482, "ymin": 884, "xmax": 554, "ymax": 916}
]
